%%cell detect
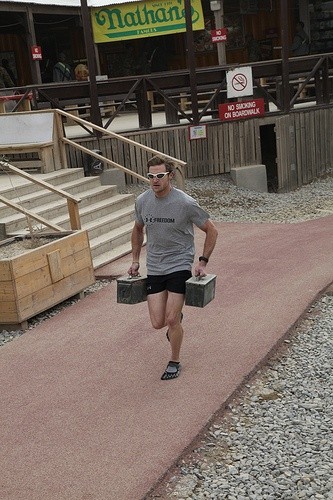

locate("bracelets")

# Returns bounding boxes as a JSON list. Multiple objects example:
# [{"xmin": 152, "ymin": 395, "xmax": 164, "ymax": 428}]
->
[
  {"xmin": 198, "ymin": 256, "xmax": 208, "ymax": 263},
  {"xmin": 131, "ymin": 261, "xmax": 140, "ymax": 265}
]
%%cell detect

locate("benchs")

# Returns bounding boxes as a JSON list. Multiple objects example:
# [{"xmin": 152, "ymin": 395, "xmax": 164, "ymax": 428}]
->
[{"xmin": 61, "ymin": 73, "xmax": 333, "ymax": 138}]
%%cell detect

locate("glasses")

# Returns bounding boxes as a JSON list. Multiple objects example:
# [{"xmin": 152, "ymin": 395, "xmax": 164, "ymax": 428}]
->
[{"xmin": 146, "ymin": 172, "xmax": 170, "ymax": 179}]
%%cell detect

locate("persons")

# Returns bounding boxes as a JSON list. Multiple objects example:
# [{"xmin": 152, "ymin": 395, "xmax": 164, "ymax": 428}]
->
[
  {"xmin": 245, "ymin": 34, "xmax": 260, "ymax": 62},
  {"xmin": 51, "ymin": 53, "xmax": 74, "ymax": 82},
  {"xmin": 289, "ymin": 21, "xmax": 311, "ymax": 56},
  {"xmin": 126, "ymin": 155, "xmax": 217, "ymax": 381}
]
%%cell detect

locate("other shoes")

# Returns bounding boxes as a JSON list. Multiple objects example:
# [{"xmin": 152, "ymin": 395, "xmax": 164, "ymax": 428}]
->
[{"xmin": 161, "ymin": 361, "xmax": 181, "ymax": 379}]
